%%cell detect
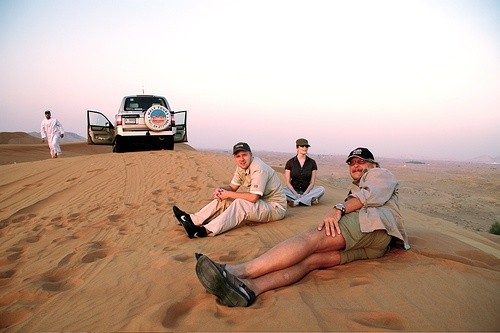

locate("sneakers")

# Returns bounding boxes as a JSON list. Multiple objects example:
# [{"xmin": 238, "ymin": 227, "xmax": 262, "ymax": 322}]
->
[
  {"xmin": 172, "ymin": 205, "xmax": 191, "ymax": 225},
  {"xmin": 290, "ymin": 199, "xmax": 297, "ymax": 207},
  {"xmin": 181, "ymin": 220, "xmax": 202, "ymax": 240},
  {"xmin": 312, "ymin": 198, "xmax": 319, "ymax": 205}
]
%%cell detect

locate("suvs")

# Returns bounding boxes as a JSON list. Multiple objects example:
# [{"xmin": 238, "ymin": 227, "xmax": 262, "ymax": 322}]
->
[{"xmin": 86, "ymin": 95, "xmax": 189, "ymax": 152}]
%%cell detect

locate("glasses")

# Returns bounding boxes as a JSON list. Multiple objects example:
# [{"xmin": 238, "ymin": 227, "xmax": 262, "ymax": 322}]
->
[{"xmin": 347, "ymin": 160, "xmax": 367, "ymax": 166}]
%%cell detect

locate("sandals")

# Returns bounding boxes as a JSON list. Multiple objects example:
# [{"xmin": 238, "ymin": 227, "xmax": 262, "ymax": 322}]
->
[{"xmin": 194, "ymin": 251, "xmax": 255, "ymax": 307}]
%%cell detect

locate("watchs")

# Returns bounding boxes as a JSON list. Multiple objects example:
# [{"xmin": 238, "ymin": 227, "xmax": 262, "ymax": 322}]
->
[{"xmin": 333, "ymin": 202, "xmax": 346, "ymax": 215}]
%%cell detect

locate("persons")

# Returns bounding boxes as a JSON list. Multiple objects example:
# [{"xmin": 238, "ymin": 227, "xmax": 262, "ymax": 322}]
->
[
  {"xmin": 283, "ymin": 138, "xmax": 325, "ymax": 207},
  {"xmin": 195, "ymin": 146, "xmax": 410, "ymax": 307},
  {"xmin": 40, "ymin": 109, "xmax": 65, "ymax": 158},
  {"xmin": 173, "ymin": 141, "xmax": 288, "ymax": 239}
]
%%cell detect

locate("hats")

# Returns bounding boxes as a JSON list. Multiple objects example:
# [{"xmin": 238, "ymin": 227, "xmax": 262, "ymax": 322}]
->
[
  {"xmin": 296, "ymin": 138, "xmax": 311, "ymax": 146},
  {"xmin": 232, "ymin": 142, "xmax": 251, "ymax": 156},
  {"xmin": 346, "ymin": 147, "xmax": 378, "ymax": 163}
]
